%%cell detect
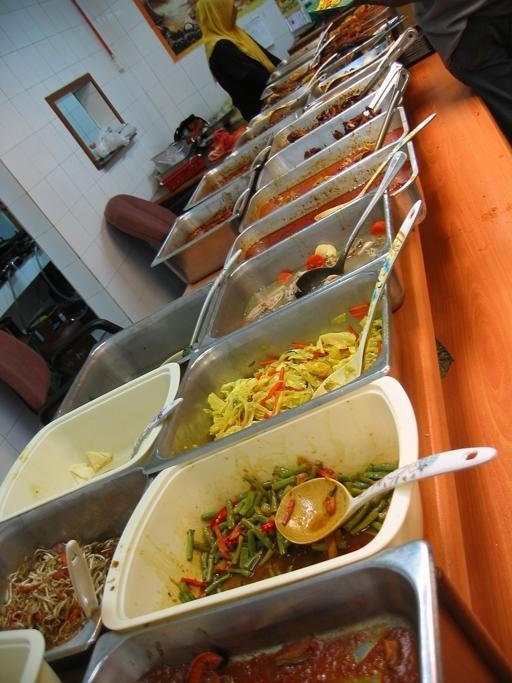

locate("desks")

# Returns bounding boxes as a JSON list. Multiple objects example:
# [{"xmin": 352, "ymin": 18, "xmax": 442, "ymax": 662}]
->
[{"xmin": 179, "ymin": 41, "xmax": 512, "ymax": 680}]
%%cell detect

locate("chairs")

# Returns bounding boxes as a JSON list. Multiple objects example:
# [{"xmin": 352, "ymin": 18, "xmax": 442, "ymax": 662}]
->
[
  {"xmin": 0, "ymin": 319, "xmax": 125, "ymax": 421},
  {"xmin": 104, "ymin": 194, "xmax": 177, "ymax": 247}
]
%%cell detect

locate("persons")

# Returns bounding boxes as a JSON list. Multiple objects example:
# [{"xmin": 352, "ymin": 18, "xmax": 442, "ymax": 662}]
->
[
  {"xmin": 196, "ymin": 0, "xmax": 282, "ymax": 120},
  {"xmin": 411, "ymin": 0, "xmax": 512, "ymax": 137}
]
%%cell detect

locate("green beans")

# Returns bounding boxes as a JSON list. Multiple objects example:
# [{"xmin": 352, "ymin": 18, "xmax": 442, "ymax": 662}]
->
[{"xmin": 176, "ymin": 454, "xmax": 398, "ymax": 603}]
